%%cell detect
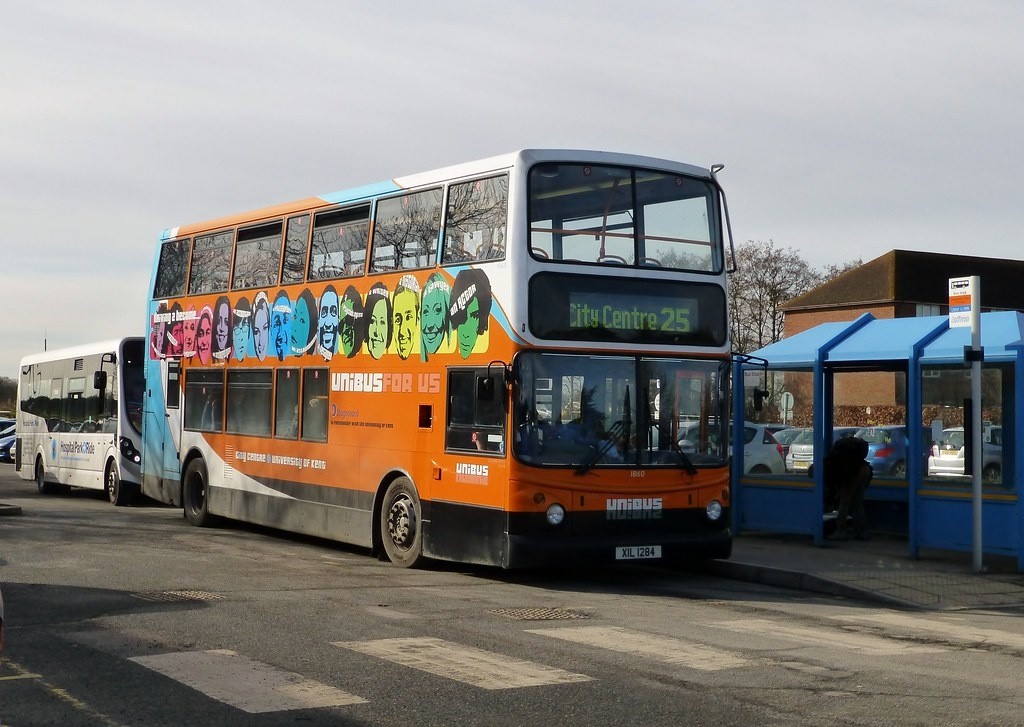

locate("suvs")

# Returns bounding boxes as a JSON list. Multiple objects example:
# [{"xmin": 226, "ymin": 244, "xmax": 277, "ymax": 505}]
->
[
  {"xmin": 853, "ymin": 424, "xmax": 933, "ymax": 478},
  {"xmin": 772, "ymin": 428, "xmax": 807, "ymax": 458},
  {"xmin": 642, "ymin": 415, "xmax": 787, "ymax": 474},
  {"xmin": 926, "ymin": 425, "xmax": 1002, "ymax": 485},
  {"xmin": 785, "ymin": 425, "xmax": 867, "ymax": 475}
]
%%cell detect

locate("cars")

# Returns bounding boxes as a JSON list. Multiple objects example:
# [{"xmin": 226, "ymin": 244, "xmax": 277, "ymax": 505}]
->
[
  {"xmin": 0, "ymin": 410, "xmax": 16, "ymax": 464},
  {"xmin": 564, "ymin": 402, "xmax": 581, "ymax": 414},
  {"xmin": 535, "ymin": 407, "xmax": 551, "ymax": 420}
]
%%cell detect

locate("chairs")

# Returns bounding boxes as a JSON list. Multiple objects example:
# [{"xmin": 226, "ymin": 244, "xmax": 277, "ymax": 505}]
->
[{"xmin": 193, "ymin": 243, "xmax": 662, "ymax": 294}]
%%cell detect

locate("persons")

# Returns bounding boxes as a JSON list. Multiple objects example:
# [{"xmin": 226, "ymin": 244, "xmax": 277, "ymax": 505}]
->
[
  {"xmin": 808, "ymin": 458, "xmax": 874, "ymax": 528},
  {"xmin": 522, "ymin": 391, "xmax": 631, "ymax": 463}
]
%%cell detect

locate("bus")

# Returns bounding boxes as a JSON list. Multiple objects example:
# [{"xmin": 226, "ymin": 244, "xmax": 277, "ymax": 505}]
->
[
  {"xmin": 139, "ymin": 146, "xmax": 769, "ymax": 573},
  {"xmin": 14, "ymin": 337, "xmax": 178, "ymax": 506}
]
